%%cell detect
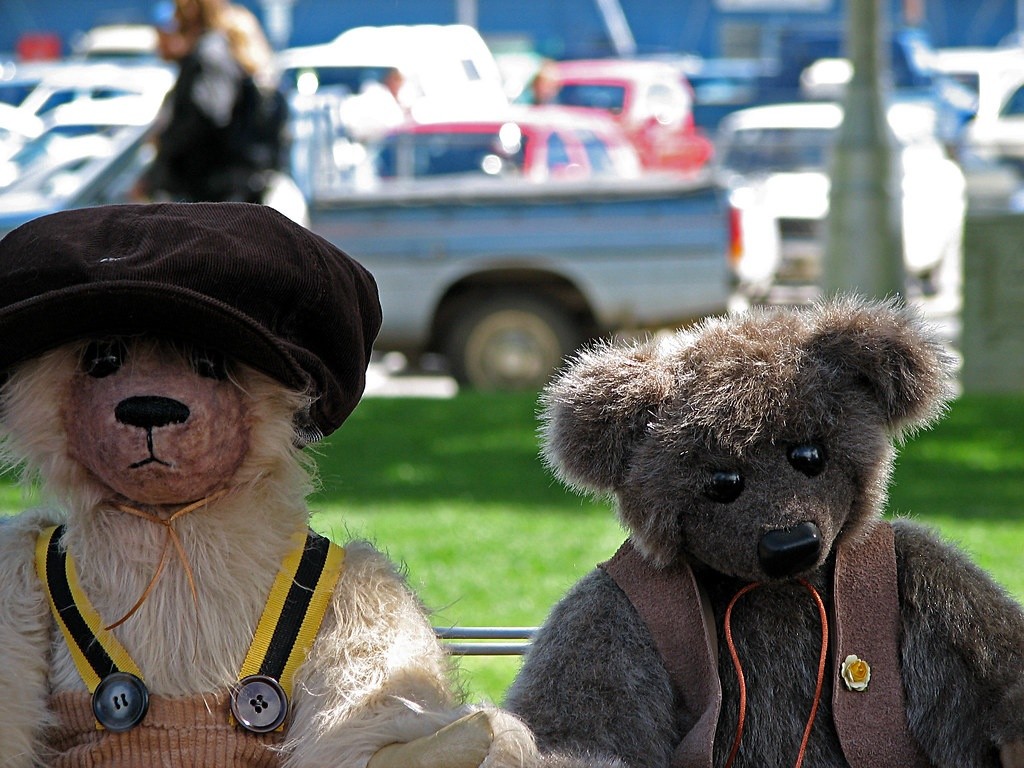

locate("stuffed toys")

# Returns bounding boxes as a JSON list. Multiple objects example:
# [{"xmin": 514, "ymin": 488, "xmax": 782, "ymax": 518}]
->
[
  {"xmin": 507, "ymin": 291, "xmax": 1024, "ymax": 768},
  {"xmin": 0, "ymin": 206, "xmax": 477, "ymax": 768}
]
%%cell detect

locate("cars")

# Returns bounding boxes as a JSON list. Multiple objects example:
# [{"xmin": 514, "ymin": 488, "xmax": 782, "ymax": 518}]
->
[{"xmin": 0, "ymin": 18, "xmax": 1024, "ymax": 396}]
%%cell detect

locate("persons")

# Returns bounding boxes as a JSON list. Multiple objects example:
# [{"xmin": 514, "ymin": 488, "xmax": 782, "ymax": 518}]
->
[
  {"xmin": 136, "ymin": 0, "xmax": 293, "ymax": 204},
  {"xmin": 889, "ymin": 0, "xmax": 945, "ymax": 92}
]
